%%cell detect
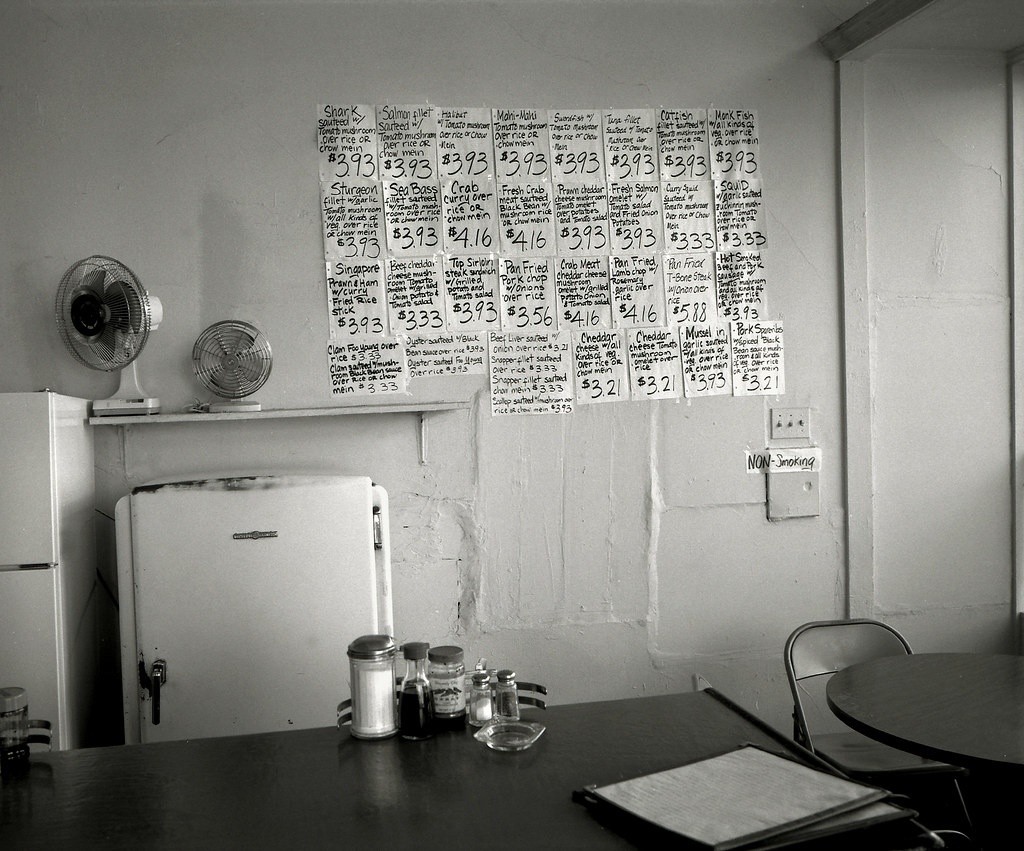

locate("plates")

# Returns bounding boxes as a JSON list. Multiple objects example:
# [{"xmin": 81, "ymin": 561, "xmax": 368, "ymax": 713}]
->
[{"xmin": 473, "ymin": 718, "xmax": 546, "ymax": 751}]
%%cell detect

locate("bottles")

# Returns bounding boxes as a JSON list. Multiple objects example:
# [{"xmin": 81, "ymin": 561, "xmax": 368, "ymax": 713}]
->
[
  {"xmin": 0, "ymin": 687, "xmax": 30, "ymax": 766},
  {"xmin": 346, "ymin": 634, "xmax": 520, "ymax": 738}
]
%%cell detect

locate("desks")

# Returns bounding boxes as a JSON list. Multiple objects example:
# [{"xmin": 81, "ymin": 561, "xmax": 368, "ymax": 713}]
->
[
  {"xmin": 0, "ymin": 681, "xmax": 931, "ymax": 849},
  {"xmin": 824, "ymin": 649, "xmax": 1024, "ymax": 850}
]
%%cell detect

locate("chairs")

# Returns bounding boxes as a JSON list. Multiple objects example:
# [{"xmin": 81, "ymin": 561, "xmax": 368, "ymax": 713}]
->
[{"xmin": 785, "ymin": 618, "xmax": 982, "ymax": 845}]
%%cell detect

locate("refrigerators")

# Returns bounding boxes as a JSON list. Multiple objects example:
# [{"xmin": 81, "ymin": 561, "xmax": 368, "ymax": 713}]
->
[{"xmin": 1, "ymin": 391, "xmax": 99, "ymax": 752}]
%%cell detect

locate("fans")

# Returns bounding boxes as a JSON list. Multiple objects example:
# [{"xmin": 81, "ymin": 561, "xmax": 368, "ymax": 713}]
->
[
  {"xmin": 51, "ymin": 257, "xmax": 169, "ymax": 419},
  {"xmin": 188, "ymin": 318, "xmax": 276, "ymax": 411}
]
